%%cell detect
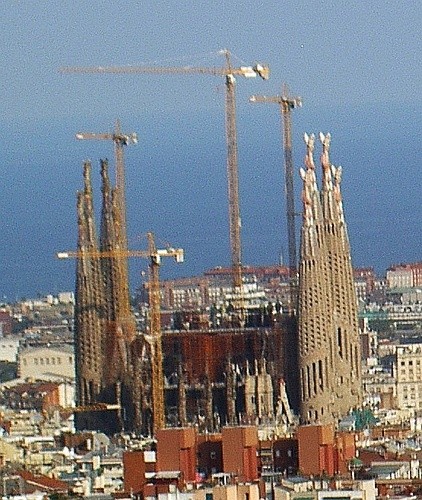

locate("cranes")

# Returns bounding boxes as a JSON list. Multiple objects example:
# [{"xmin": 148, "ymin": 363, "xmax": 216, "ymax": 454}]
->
[
  {"xmin": 247, "ymin": 83, "xmax": 304, "ymax": 316},
  {"xmin": 73, "ymin": 117, "xmax": 136, "ymax": 327},
  {"xmin": 54, "ymin": 232, "xmax": 185, "ymax": 434},
  {"xmin": 53, "ymin": 47, "xmax": 269, "ymax": 323}
]
